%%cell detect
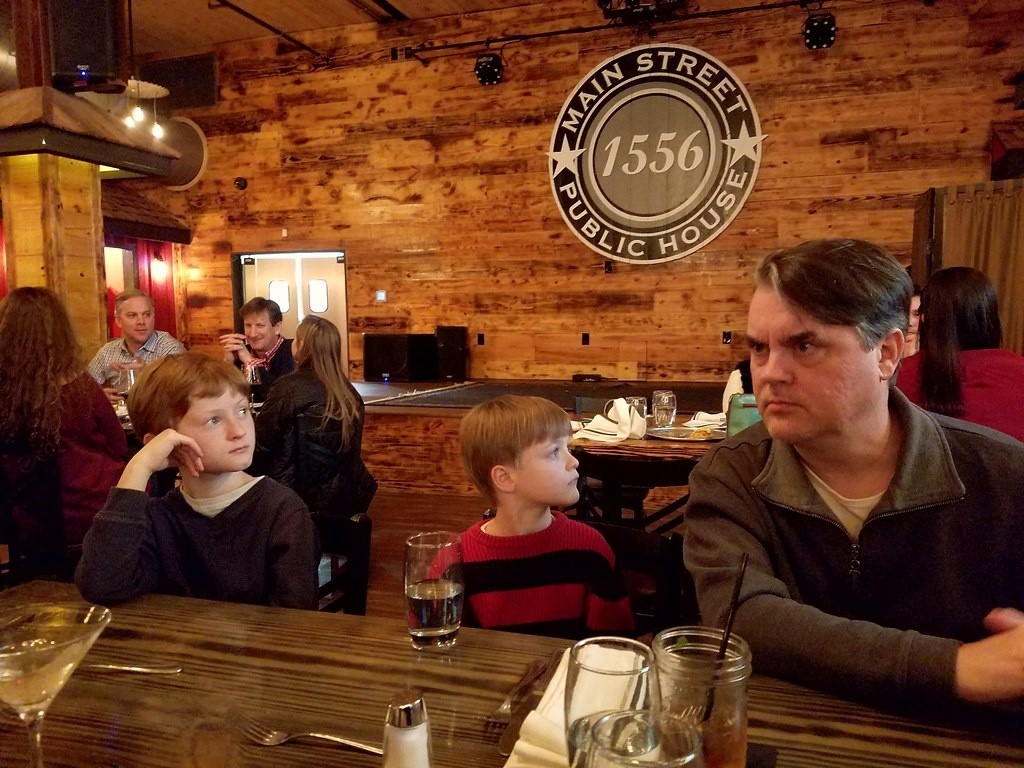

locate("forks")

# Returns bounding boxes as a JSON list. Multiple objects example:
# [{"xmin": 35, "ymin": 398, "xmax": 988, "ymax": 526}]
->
[
  {"xmin": 229, "ymin": 708, "xmax": 383, "ymax": 755},
  {"xmin": 483, "ymin": 659, "xmax": 546, "ymax": 730}
]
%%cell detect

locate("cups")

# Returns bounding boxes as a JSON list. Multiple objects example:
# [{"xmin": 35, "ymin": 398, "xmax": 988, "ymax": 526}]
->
[
  {"xmin": 564, "ymin": 635, "xmax": 662, "ymax": 768},
  {"xmin": 652, "ymin": 625, "xmax": 752, "ymax": 768},
  {"xmin": 403, "ymin": 530, "xmax": 464, "ymax": 650},
  {"xmin": 654, "ymin": 395, "xmax": 677, "ymax": 428},
  {"xmin": 585, "ymin": 709, "xmax": 705, "ymax": 768},
  {"xmin": 118, "ymin": 368, "xmax": 135, "ymax": 398},
  {"xmin": 652, "ymin": 390, "xmax": 673, "ymax": 421},
  {"xmin": 626, "ymin": 396, "xmax": 647, "ymax": 419}
]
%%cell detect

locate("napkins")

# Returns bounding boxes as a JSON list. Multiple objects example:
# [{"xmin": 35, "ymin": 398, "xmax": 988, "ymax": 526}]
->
[
  {"xmin": 573, "ymin": 397, "xmax": 647, "ymax": 441},
  {"xmin": 570, "ymin": 420, "xmax": 590, "ymax": 430},
  {"xmin": 505, "ymin": 643, "xmax": 647, "ymax": 768},
  {"xmin": 684, "ymin": 411, "xmax": 727, "ymax": 429}
]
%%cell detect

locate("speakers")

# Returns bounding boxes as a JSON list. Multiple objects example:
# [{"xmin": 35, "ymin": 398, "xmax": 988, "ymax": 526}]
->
[
  {"xmin": 360, "ymin": 332, "xmax": 437, "ymax": 383},
  {"xmin": 433, "ymin": 326, "xmax": 478, "ymax": 387},
  {"xmin": 46, "ymin": 0, "xmax": 129, "ymax": 95}
]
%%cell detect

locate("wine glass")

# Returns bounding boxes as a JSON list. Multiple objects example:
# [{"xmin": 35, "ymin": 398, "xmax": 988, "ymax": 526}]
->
[
  {"xmin": 240, "ymin": 363, "xmax": 262, "ymax": 413},
  {"xmin": 0, "ymin": 599, "xmax": 112, "ymax": 768}
]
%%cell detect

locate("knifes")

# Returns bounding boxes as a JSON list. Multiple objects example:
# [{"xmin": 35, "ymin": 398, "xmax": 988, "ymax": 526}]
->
[{"xmin": 497, "ymin": 647, "xmax": 564, "ymax": 757}]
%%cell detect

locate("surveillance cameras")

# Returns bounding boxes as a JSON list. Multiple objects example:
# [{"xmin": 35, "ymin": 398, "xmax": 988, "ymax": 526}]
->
[{"xmin": 234, "ymin": 177, "xmax": 247, "ymax": 190}]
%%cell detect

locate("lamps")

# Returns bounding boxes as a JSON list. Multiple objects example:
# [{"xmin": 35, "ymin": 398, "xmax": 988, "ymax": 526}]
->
[
  {"xmin": 803, "ymin": 13, "xmax": 837, "ymax": 50},
  {"xmin": 120, "ymin": 0, "xmax": 168, "ymax": 140},
  {"xmin": 474, "ymin": 54, "xmax": 505, "ymax": 85}
]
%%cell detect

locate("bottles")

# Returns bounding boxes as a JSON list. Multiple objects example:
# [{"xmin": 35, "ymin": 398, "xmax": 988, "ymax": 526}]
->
[{"xmin": 382, "ymin": 688, "xmax": 435, "ymax": 768}]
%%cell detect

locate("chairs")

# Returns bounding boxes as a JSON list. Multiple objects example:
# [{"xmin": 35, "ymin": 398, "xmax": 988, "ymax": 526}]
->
[
  {"xmin": 0, "ymin": 437, "xmax": 84, "ymax": 579},
  {"xmin": 291, "ymin": 406, "xmax": 702, "ymax": 644}
]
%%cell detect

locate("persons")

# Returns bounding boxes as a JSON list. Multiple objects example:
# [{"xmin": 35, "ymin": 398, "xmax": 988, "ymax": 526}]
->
[
  {"xmin": 722, "ymin": 359, "xmax": 753, "ymax": 414},
  {"xmin": 0, "ymin": 287, "xmax": 152, "ymax": 579},
  {"xmin": 896, "ymin": 266, "xmax": 1024, "ymax": 443},
  {"xmin": 254, "ymin": 317, "xmax": 377, "ymax": 517},
  {"xmin": 409, "ymin": 394, "xmax": 635, "ymax": 640},
  {"xmin": 219, "ymin": 297, "xmax": 297, "ymax": 412},
  {"xmin": 88, "ymin": 289, "xmax": 186, "ymax": 405},
  {"xmin": 75, "ymin": 351, "xmax": 317, "ymax": 611},
  {"xmin": 682, "ymin": 239, "xmax": 1024, "ymax": 709}
]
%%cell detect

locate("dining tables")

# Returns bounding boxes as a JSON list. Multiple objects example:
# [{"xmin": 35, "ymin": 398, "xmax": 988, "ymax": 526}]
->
[
  {"xmin": 0, "ymin": 575, "xmax": 1024, "ymax": 768},
  {"xmin": 566, "ymin": 411, "xmax": 731, "ymax": 528}
]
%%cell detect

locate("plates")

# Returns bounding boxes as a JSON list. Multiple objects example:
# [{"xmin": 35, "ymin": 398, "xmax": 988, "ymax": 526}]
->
[{"xmin": 646, "ymin": 427, "xmax": 726, "ymax": 441}]
